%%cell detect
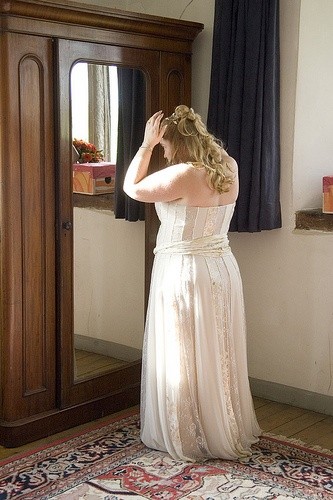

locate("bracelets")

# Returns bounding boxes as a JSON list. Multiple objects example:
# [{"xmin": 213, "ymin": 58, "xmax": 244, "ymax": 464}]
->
[{"xmin": 139, "ymin": 142, "xmax": 154, "ymax": 152}]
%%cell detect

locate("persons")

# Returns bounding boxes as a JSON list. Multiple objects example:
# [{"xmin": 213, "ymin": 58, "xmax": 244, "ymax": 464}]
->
[{"xmin": 123, "ymin": 105, "xmax": 264, "ymax": 463}]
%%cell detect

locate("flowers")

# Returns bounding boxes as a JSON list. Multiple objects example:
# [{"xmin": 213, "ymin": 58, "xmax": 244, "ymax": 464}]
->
[{"xmin": 74, "ymin": 138, "xmax": 103, "ymax": 163}]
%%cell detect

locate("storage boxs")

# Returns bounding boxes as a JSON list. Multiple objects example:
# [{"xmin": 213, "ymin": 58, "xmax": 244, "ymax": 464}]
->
[
  {"xmin": 73, "ymin": 162, "xmax": 116, "ymax": 195},
  {"xmin": 322, "ymin": 175, "xmax": 333, "ymax": 214}
]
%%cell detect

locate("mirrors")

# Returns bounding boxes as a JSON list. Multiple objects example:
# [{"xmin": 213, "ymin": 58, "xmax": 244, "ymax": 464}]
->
[{"xmin": 69, "ymin": 58, "xmax": 148, "ymax": 387}]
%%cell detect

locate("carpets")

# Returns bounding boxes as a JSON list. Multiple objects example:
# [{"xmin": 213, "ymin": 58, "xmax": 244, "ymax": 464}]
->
[{"xmin": 0, "ymin": 410, "xmax": 333, "ymax": 500}]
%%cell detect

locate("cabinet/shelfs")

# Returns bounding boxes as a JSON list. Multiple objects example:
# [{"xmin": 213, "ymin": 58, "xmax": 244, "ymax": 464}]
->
[{"xmin": 0, "ymin": 0, "xmax": 204, "ymax": 449}]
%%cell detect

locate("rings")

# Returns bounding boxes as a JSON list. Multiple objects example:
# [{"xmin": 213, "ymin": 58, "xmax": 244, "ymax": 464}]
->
[{"xmin": 148, "ymin": 121, "xmax": 150, "ymax": 124}]
